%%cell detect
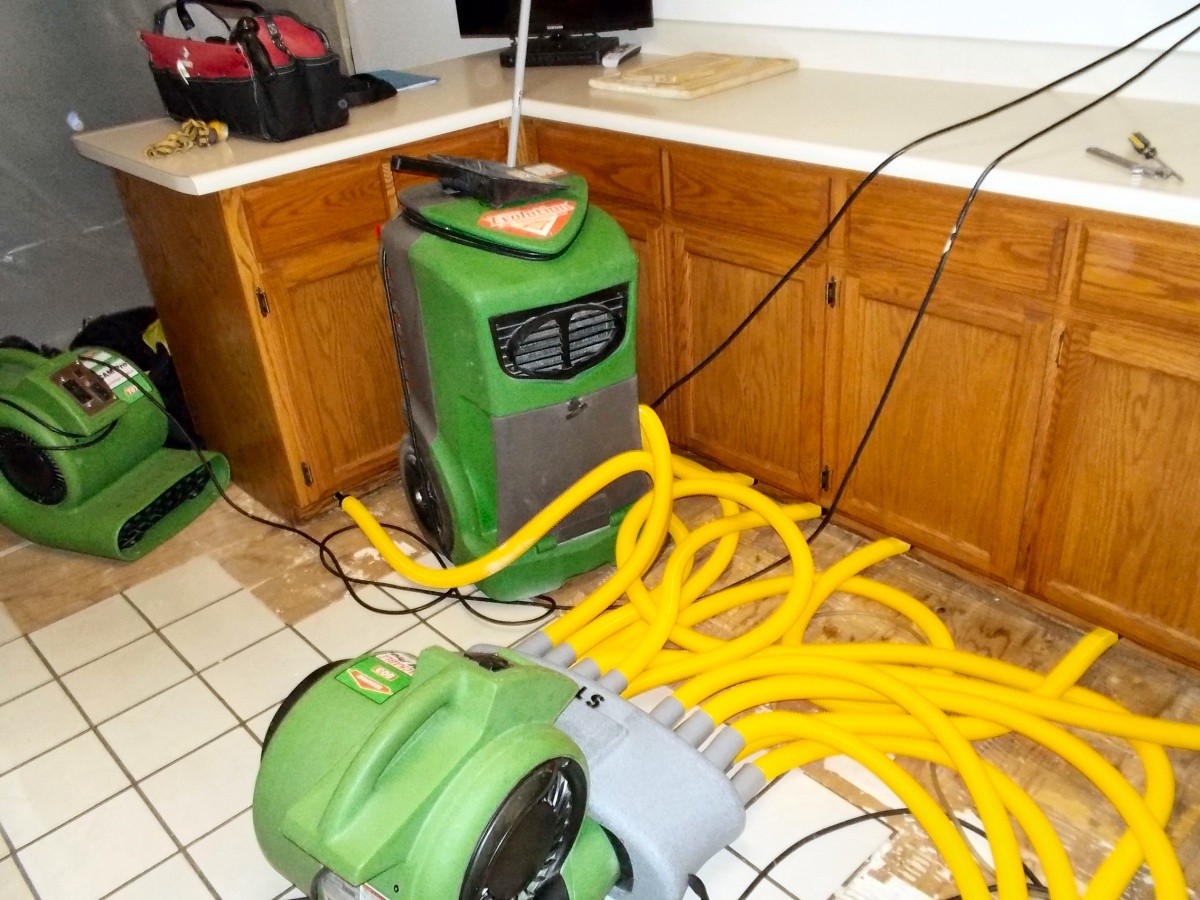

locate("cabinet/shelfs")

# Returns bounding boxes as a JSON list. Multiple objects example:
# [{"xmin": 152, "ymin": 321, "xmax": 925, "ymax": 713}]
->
[{"xmin": 111, "ymin": 117, "xmax": 1200, "ymax": 691}]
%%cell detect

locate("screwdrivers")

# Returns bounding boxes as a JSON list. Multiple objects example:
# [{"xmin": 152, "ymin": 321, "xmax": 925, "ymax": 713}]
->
[{"xmin": 1129, "ymin": 130, "xmax": 1185, "ymax": 183}]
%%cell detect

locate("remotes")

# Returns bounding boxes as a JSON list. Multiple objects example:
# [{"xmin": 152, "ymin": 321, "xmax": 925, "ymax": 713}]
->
[{"xmin": 602, "ymin": 43, "xmax": 641, "ymax": 68}]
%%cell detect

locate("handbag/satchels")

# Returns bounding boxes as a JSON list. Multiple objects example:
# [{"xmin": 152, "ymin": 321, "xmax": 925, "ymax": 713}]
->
[{"xmin": 136, "ymin": 0, "xmax": 350, "ymax": 142}]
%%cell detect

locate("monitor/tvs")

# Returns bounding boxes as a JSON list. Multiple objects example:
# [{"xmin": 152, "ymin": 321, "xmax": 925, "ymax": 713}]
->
[{"xmin": 455, "ymin": 0, "xmax": 654, "ymax": 52}]
